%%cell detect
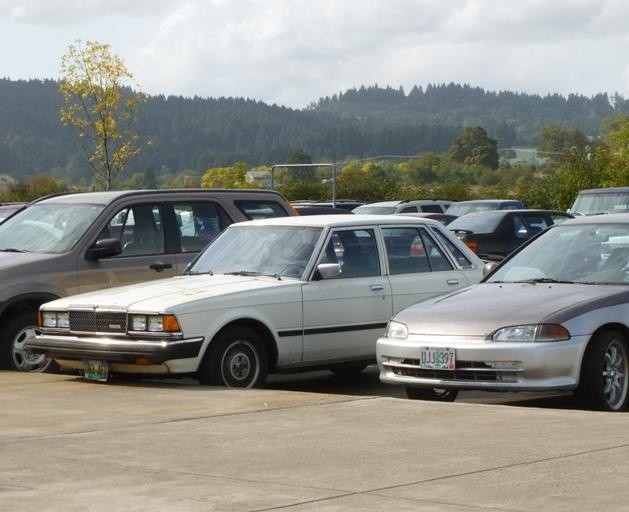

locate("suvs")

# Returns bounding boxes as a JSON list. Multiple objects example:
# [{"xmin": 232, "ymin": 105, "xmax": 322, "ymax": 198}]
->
[{"xmin": 0, "ymin": 188, "xmax": 304, "ymax": 373}]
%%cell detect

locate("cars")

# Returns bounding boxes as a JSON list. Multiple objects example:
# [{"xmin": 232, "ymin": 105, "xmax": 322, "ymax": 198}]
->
[
  {"xmin": 372, "ymin": 216, "xmax": 629, "ymax": 413},
  {"xmin": 17, "ymin": 213, "xmax": 550, "ymax": 391}
]
